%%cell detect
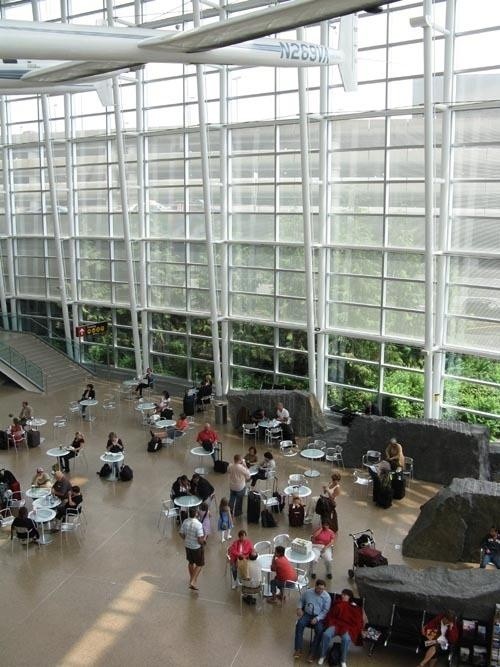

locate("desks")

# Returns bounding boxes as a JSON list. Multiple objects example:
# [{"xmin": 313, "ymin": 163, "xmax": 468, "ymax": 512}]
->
[
  {"xmin": 191, "ymin": 447, "xmax": 214, "ymax": 475},
  {"xmin": 80, "ymin": 400, "xmax": 98, "ymax": 422},
  {"xmin": 46, "ymin": 447, "xmax": 70, "ymax": 472},
  {"xmin": 100, "ymin": 453, "xmax": 125, "ymax": 482}
]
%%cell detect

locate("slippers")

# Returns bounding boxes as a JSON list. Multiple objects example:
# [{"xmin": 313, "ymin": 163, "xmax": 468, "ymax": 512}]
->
[{"xmin": 190, "ymin": 584, "xmax": 199, "ymax": 590}]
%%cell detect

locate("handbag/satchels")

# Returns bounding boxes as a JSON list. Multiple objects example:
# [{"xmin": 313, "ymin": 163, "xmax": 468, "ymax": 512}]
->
[
  {"xmin": 261, "ymin": 510, "xmax": 275, "ymax": 527},
  {"xmin": 147, "ymin": 437, "xmax": 162, "ymax": 452},
  {"xmin": 202, "ymin": 439, "xmax": 213, "ymax": 452},
  {"xmin": 99, "ymin": 463, "xmax": 111, "ymax": 477},
  {"xmin": 120, "ymin": 465, "xmax": 133, "ymax": 481},
  {"xmin": 327, "ymin": 641, "xmax": 343, "ymax": 666},
  {"xmin": 316, "ymin": 496, "xmax": 336, "ymax": 515}
]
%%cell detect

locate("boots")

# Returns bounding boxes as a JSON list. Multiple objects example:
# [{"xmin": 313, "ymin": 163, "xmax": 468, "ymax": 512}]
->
[
  {"xmin": 227, "ymin": 529, "xmax": 232, "ymax": 539},
  {"xmin": 221, "ymin": 531, "xmax": 226, "ymax": 542}
]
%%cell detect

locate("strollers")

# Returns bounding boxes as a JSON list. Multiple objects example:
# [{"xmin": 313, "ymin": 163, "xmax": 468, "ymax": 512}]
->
[{"xmin": 347, "ymin": 529, "xmax": 390, "ymax": 581}]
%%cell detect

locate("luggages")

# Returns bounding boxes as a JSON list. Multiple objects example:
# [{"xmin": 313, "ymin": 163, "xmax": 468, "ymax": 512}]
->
[
  {"xmin": 26, "ymin": 428, "xmax": 41, "ymax": 448},
  {"xmin": 183, "ymin": 391, "xmax": 194, "ymax": 416},
  {"xmin": 271, "ymin": 477, "xmax": 285, "ymax": 513},
  {"xmin": 214, "ymin": 442, "xmax": 229, "ymax": 474},
  {"xmin": 247, "ymin": 491, "xmax": 261, "ymax": 524},
  {"xmin": 391, "ymin": 465, "xmax": 406, "ymax": 500},
  {"xmin": 289, "ymin": 486, "xmax": 305, "ymax": 527},
  {"xmin": 356, "ymin": 544, "xmax": 389, "ymax": 567}
]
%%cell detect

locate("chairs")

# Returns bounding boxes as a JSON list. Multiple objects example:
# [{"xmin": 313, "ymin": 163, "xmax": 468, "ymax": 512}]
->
[
  {"xmin": 1, "ymin": 416, "xmax": 48, "ymax": 453},
  {"xmin": 119, "ymin": 379, "xmax": 216, "ymax": 446},
  {"xmin": 102, "ymin": 388, "xmax": 119, "ymax": 415},
  {"xmin": 157, "ymin": 491, "xmax": 226, "ymax": 544},
  {"xmin": 384, "ymin": 604, "xmax": 457, "ymax": 666},
  {"xmin": 0, "ymin": 475, "xmax": 86, "ymax": 557},
  {"xmin": 223, "ymin": 534, "xmax": 333, "ymax": 616},
  {"xmin": 240, "ymin": 417, "xmax": 345, "ymax": 524},
  {"xmin": 53, "ymin": 399, "xmax": 81, "ymax": 433},
  {"xmin": 299, "ymin": 594, "xmax": 365, "ymax": 662},
  {"xmin": 63, "ymin": 444, "xmax": 88, "ymax": 469},
  {"xmin": 352, "ymin": 451, "xmax": 414, "ymax": 501}
]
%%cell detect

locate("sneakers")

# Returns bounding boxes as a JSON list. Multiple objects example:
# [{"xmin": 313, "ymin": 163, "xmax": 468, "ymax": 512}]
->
[
  {"xmin": 312, "ymin": 573, "xmax": 332, "ymax": 579},
  {"xmin": 232, "ymin": 580, "xmax": 287, "ymax": 605},
  {"xmin": 293, "ymin": 650, "xmax": 347, "ymax": 666}
]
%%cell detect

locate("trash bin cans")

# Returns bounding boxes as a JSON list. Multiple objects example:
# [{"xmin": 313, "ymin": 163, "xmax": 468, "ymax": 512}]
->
[{"xmin": 214, "ymin": 403, "xmax": 228, "ymax": 425}]
